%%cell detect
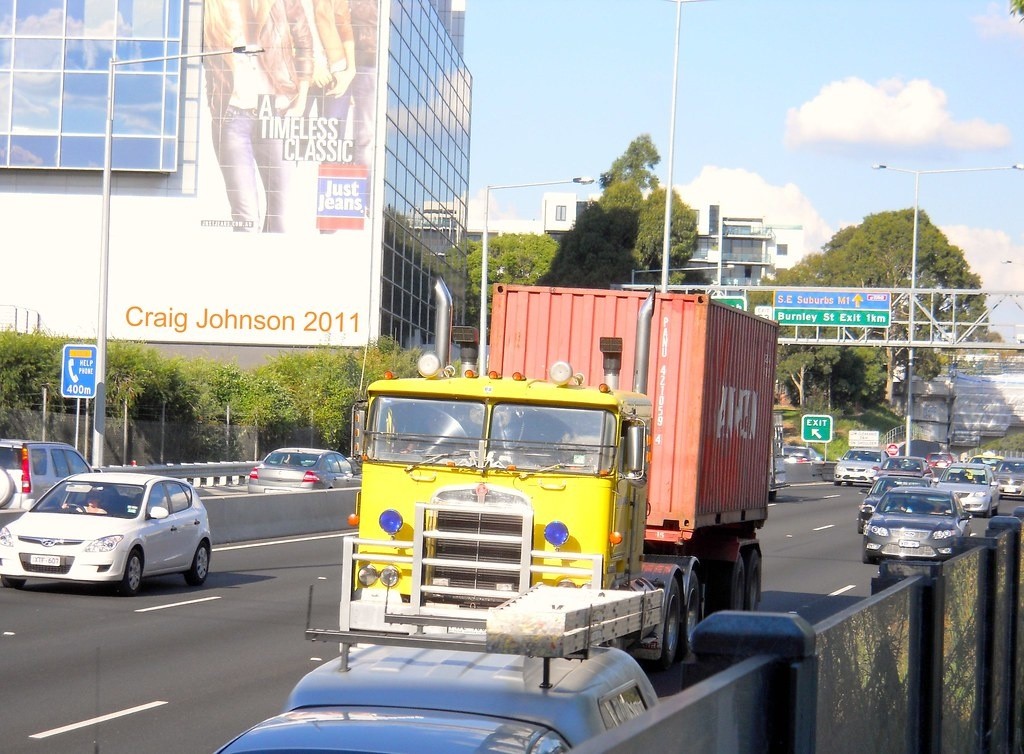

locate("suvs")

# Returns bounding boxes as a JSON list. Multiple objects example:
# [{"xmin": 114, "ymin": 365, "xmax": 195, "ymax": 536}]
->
[
  {"xmin": 925, "ymin": 452, "xmax": 960, "ymax": 469},
  {"xmin": 0, "ymin": 438, "xmax": 102, "ymax": 510}
]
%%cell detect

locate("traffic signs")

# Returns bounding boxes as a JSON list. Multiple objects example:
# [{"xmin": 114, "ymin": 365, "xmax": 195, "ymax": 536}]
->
[
  {"xmin": 801, "ymin": 414, "xmax": 833, "ymax": 444},
  {"xmin": 772, "ymin": 288, "xmax": 891, "ymax": 329}
]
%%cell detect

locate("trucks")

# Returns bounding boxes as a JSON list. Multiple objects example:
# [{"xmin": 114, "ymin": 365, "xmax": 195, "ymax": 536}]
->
[{"xmin": 768, "ymin": 409, "xmax": 790, "ymax": 500}]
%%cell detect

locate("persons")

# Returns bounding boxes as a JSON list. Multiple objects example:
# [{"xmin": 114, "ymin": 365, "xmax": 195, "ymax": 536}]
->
[
  {"xmin": 1003, "ymin": 463, "xmax": 1012, "ymax": 472},
  {"xmin": 925, "ymin": 501, "xmax": 945, "ymax": 514},
  {"xmin": 203, "ymin": 0, "xmax": 377, "ymax": 233},
  {"xmin": 954, "ymin": 474, "xmax": 963, "ymax": 480},
  {"xmin": 885, "ymin": 498, "xmax": 913, "ymax": 512},
  {"xmin": 971, "ymin": 474, "xmax": 982, "ymax": 484},
  {"xmin": 62, "ymin": 492, "xmax": 108, "ymax": 514}
]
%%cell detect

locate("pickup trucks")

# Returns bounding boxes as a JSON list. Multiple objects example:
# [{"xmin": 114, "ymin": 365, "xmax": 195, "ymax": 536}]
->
[{"xmin": 205, "ymin": 643, "xmax": 660, "ymax": 754}]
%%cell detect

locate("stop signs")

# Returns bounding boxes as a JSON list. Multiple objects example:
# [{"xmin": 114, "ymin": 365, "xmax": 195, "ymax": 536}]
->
[{"xmin": 886, "ymin": 443, "xmax": 899, "ymax": 457}]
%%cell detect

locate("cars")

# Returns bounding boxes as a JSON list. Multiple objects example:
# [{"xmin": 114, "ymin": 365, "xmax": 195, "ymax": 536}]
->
[
  {"xmin": 861, "ymin": 485, "xmax": 972, "ymax": 565},
  {"xmin": 783, "ymin": 446, "xmax": 820, "ymax": 462},
  {"xmin": 246, "ymin": 446, "xmax": 362, "ymax": 495},
  {"xmin": 965, "ymin": 451, "xmax": 1005, "ymax": 479},
  {"xmin": 872, "ymin": 455, "xmax": 934, "ymax": 487},
  {"xmin": 0, "ymin": 473, "xmax": 212, "ymax": 597},
  {"xmin": 834, "ymin": 447, "xmax": 890, "ymax": 487},
  {"xmin": 858, "ymin": 476, "xmax": 933, "ymax": 534},
  {"xmin": 994, "ymin": 459, "xmax": 1024, "ymax": 500},
  {"xmin": 932, "ymin": 462, "xmax": 1000, "ymax": 518}
]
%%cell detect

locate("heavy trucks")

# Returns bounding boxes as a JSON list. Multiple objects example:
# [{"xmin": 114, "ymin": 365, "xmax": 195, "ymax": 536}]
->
[{"xmin": 338, "ymin": 283, "xmax": 783, "ymax": 689}]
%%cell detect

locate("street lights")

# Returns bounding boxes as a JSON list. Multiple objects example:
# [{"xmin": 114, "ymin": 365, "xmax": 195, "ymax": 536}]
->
[
  {"xmin": 478, "ymin": 176, "xmax": 595, "ymax": 375},
  {"xmin": 870, "ymin": 161, "xmax": 1024, "ymax": 470},
  {"xmin": 89, "ymin": 43, "xmax": 266, "ymax": 467},
  {"xmin": 632, "ymin": 263, "xmax": 736, "ymax": 285}
]
